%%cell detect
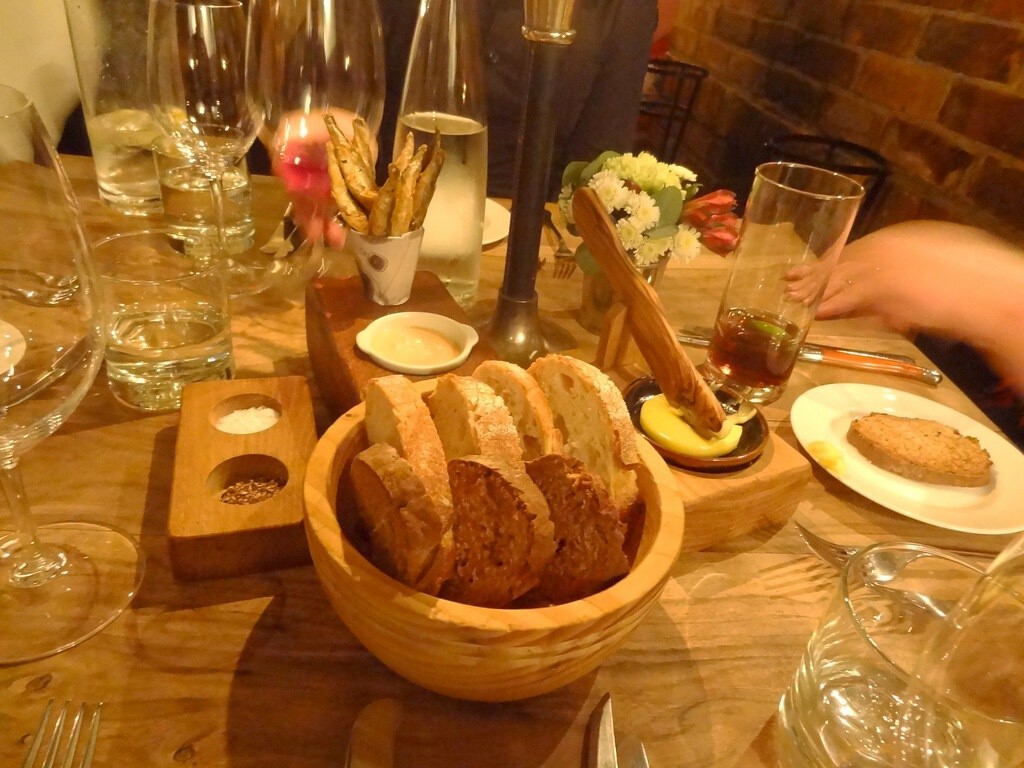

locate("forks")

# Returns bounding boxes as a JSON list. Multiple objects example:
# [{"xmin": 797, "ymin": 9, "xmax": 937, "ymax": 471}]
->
[
  {"xmin": 21, "ymin": 697, "xmax": 104, "ymax": 768},
  {"xmin": 793, "ymin": 518, "xmax": 1001, "ymax": 574},
  {"xmin": 543, "ymin": 209, "xmax": 576, "ymax": 277}
]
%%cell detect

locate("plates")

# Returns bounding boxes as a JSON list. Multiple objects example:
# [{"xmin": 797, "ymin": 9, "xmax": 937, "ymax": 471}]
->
[
  {"xmin": 481, "ymin": 198, "xmax": 510, "ymax": 245},
  {"xmin": 790, "ymin": 383, "xmax": 1024, "ymax": 534}
]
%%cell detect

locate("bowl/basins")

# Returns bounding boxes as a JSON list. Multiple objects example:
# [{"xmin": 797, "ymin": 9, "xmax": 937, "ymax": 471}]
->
[
  {"xmin": 621, "ymin": 374, "xmax": 768, "ymax": 472},
  {"xmin": 304, "ymin": 376, "xmax": 685, "ymax": 702},
  {"xmin": 356, "ymin": 311, "xmax": 479, "ymax": 376}
]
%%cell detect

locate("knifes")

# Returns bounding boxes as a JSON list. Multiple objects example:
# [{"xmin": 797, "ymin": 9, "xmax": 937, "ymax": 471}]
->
[
  {"xmin": 585, "ymin": 692, "xmax": 618, "ymax": 768},
  {"xmin": 676, "ymin": 334, "xmax": 942, "ymax": 384}
]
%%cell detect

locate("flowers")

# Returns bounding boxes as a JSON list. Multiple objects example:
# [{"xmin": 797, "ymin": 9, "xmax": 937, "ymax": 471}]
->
[{"xmin": 558, "ymin": 148, "xmax": 701, "ymax": 270}]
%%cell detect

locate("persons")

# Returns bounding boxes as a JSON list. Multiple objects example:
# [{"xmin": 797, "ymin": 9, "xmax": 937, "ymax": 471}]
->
[
  {"xmin": 377, "ymin": 0, "xmax": 659, "ymax": 198},
  {"xmin": 787, "ymin": 219, "xmax": 1024, "ymax": 413}
]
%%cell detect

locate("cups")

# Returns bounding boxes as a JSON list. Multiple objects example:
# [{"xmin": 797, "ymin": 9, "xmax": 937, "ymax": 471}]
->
[
  {"xmin": 76, "ymin": 227, "xmax": 235, "ymax": 410},
  {"xmin": 345, "ymin": 225, "xmax": 423, "ymax": 305},
  {"xmin": 773, "ymin": 539, "xmax": 1024, "ymax": 768},
  {"xmin": 704, "ymin": 161, "xmax": 866, "ymax": 404},
  {"xmin": 64, "ymin": 0, "xmax": 187, "ymax": 216},
  {"xmin": 150, "ymin": 124, "xmax": 250, "ymax": 258}
]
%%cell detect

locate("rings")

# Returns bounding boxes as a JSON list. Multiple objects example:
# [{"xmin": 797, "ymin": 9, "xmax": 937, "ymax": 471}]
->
[{"xmin": 846, "ymin": 279, "xmax": 854, "ymax": 286}]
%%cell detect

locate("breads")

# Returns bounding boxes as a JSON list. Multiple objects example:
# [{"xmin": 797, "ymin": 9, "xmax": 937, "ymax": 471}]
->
[
  {"xmin": 346, "ymin": 353, "xmax": 641, "ymax": 608},
  {"xmin": 847, "ymin": 412, "xmax": 992, "ymax": 488}
]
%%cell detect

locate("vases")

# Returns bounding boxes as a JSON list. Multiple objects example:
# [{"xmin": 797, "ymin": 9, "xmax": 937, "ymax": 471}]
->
[{"xmin": 576, "ymin": 234, "xmax": 671, "ymax": 337}]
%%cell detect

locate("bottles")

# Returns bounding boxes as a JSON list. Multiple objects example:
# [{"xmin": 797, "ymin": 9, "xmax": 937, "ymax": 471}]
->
[{"xmin": 393, "ymin": 0, "xmax": 487, "ymax": 312}]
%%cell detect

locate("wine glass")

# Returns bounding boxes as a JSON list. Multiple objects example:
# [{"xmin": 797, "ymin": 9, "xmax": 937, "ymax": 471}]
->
[
  {"xmin": 0, "ymin": 83, "xmax": 145, "ymax": 666},
  {"xmin": 245, "ymin": 0, "xmax": 384, "ymax": 305},
  {"xmin": 147, "ymin": 0, "xmax": 274, "ymax": 252}
]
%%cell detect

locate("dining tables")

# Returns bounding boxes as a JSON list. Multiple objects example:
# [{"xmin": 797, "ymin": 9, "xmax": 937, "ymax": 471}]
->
[{"xmin": 0, "ymin": 152, "xmax": 1024, "ymax": 768}]
